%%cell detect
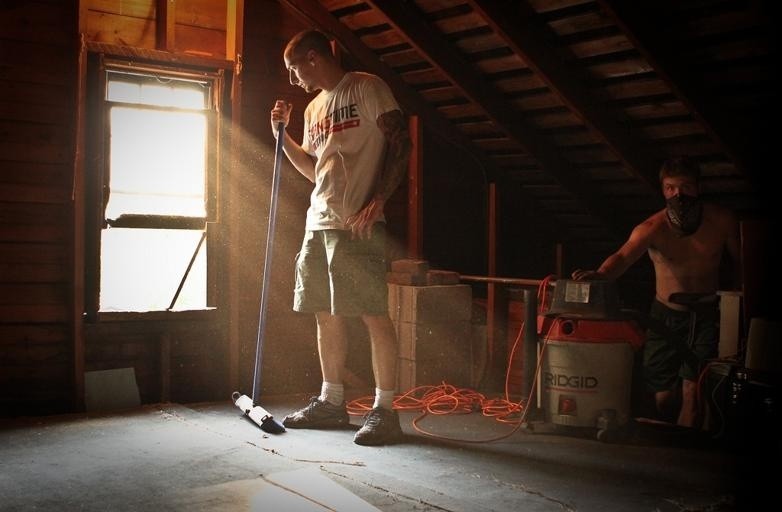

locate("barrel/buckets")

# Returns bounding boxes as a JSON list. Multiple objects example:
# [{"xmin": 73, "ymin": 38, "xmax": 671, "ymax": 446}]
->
[{"xmin": 535, "ymin": 314, "xmax": 636, "ymax": 430}]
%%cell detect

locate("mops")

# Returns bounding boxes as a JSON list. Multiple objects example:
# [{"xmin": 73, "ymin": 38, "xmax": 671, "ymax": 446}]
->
[{"xmin": 233, "ymin": 121, "xmax": 284, "ymax": 435}]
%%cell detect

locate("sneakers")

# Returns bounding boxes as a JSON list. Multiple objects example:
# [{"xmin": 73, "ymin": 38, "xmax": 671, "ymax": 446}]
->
[
  {"xmin": 354, "ymin": 407, "xmax": 403, "ymax": 446},
  {"xmin": 282, "ymin": 397, "xmax": 350, "ymax": 429}
]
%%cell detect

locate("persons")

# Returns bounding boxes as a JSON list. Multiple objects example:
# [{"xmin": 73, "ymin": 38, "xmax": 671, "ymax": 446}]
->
[
  {"xmin": 570, "ymin": 150, "xmax": 740, "ymax": 421},
  {"xmin": 270, "ymin": 31, "xmax": 412, "ymax": 447}
]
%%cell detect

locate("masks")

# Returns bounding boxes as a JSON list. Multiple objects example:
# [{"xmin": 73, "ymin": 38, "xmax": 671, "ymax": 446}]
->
[{"xmin": 664, "ymin": 194, "xmax": 701, "ymax": 230}]
